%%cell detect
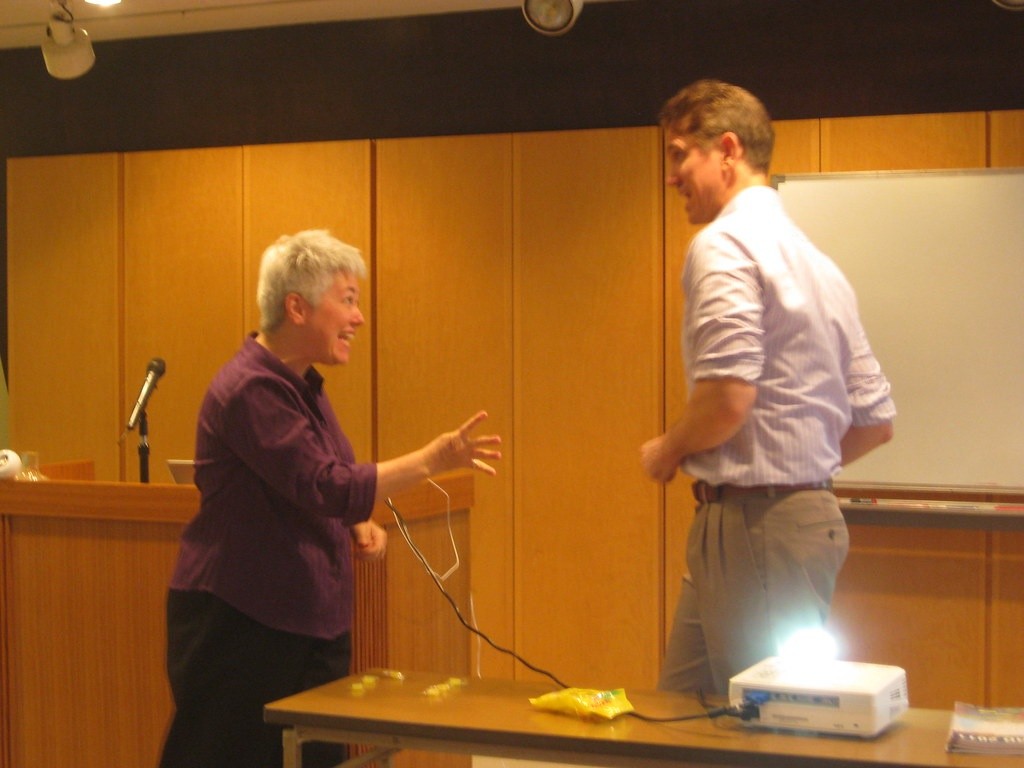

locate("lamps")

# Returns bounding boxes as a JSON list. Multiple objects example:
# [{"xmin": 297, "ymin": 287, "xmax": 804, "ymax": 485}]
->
[
  {"xmin": 522, "ymin": 0, "xmax": 584, "ymax": 36},
  {"xmin": 41, "ymin": 0, "xmax": 96, "ymax": 80}
]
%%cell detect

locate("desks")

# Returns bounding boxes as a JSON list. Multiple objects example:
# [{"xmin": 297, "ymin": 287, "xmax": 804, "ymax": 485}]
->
[{"xmin": 265, "ymin": 670, "xmax": 1024, "ymax": 768}]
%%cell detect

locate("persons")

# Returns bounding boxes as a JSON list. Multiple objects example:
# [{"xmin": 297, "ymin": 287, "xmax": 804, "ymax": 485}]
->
[
  {"xmin": 641, "ymin": 80, "xmax": 895, "ymax": 700},
  {"xmin": 157, "ymin": 231, "xmax": 501, "ymax": 768}
]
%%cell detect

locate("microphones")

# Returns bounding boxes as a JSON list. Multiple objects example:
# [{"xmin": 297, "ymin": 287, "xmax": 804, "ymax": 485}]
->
[{"xmin": 128, "ymin": 358, "xmax": 165, "ymax": 431}]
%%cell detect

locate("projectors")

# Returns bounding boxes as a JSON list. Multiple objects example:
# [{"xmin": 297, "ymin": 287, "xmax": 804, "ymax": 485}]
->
[{"xmin": 729, "ymin": 654, "xmax": 910, "ymax": 736}]
